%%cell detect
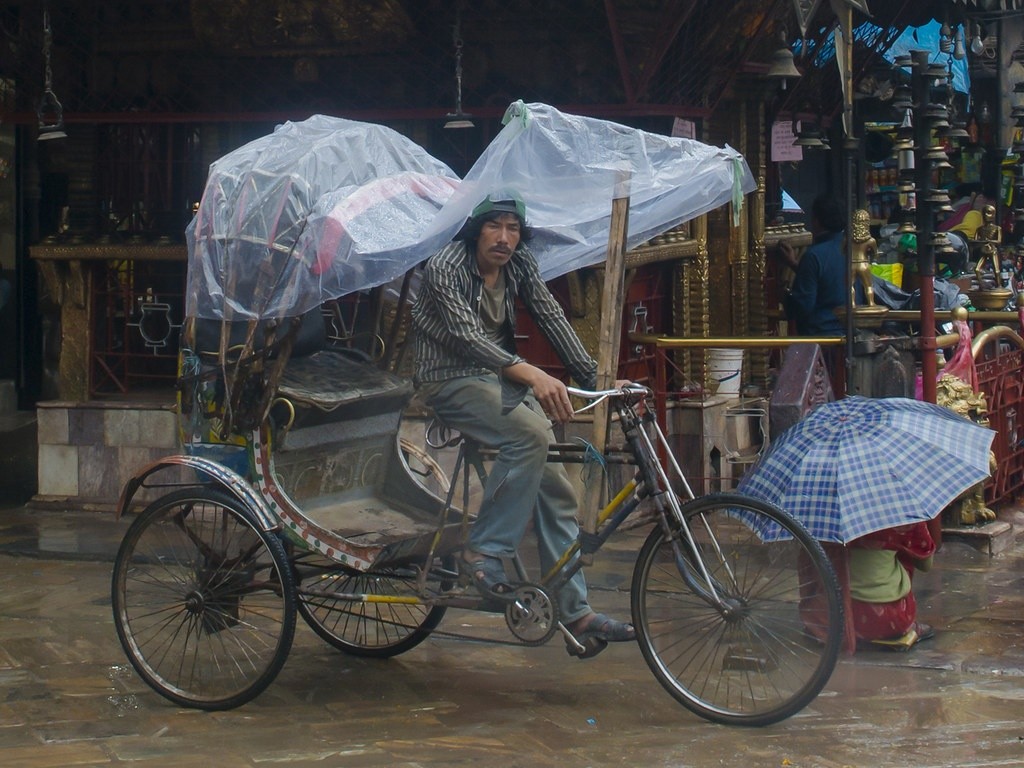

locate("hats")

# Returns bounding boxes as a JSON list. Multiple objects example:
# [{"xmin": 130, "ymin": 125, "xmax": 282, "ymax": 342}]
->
[{"xmin": 471, "ymin": 186, "xmax": 526, "ymax": 220}]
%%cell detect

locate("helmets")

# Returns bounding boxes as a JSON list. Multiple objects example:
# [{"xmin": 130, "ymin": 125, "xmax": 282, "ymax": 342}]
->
[{"xmin": 933, "ymin": 231, "xmax": 969, "ymax": 279}]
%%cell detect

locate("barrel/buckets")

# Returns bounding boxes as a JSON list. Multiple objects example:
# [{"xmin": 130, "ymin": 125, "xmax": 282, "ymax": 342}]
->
[{"xmin": 708, "ymin": 346, "xmax": 745, "ymax": 399}]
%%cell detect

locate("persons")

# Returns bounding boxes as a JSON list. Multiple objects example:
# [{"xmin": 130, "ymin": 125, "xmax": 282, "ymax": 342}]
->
[
  {"xmin": 974, "ymin": 205, "xmax": 1004, "ymax": 291},
  {"xmin": 843, "ymin": 209, "xmax": 879, "ymax": 308},
  {"xmin": 409, "ymin": 187, "xmax": 639, "ymax": 657},
  {"xmin": 778, "ymin": 191, "xmax": 864, "ymax": 403},
  {"xmin": 796, "ymin": 522, "xmax": 935, "ymax": 651}
]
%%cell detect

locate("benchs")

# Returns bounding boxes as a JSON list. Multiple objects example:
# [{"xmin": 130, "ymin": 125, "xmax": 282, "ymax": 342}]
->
[{"xmin": 181, "ymin": 292, "xmax": 421, "ymax": 454}]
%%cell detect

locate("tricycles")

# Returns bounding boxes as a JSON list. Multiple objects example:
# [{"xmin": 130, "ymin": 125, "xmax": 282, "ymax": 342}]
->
[{"xmin": 102, "ymin": 96, "xmax": 851, "ymax": 728}]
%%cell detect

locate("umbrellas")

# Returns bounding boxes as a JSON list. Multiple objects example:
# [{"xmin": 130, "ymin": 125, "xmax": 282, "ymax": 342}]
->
[{"xmin": 723, "ymin": 393, "xmax": 1000, "ymax": 547}]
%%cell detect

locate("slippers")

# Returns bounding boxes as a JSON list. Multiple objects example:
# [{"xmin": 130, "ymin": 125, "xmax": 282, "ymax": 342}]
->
[
  {"xmin": 457, "ymin": 554, "xmax": 518, "ymax": 604},
  {"xmin": 901, "ymin": 622, "xmax": 934, "ymax": 643},
  {"xmin": 564, "ymin": 613, "xmax": 636, "ymax": 657}
]
